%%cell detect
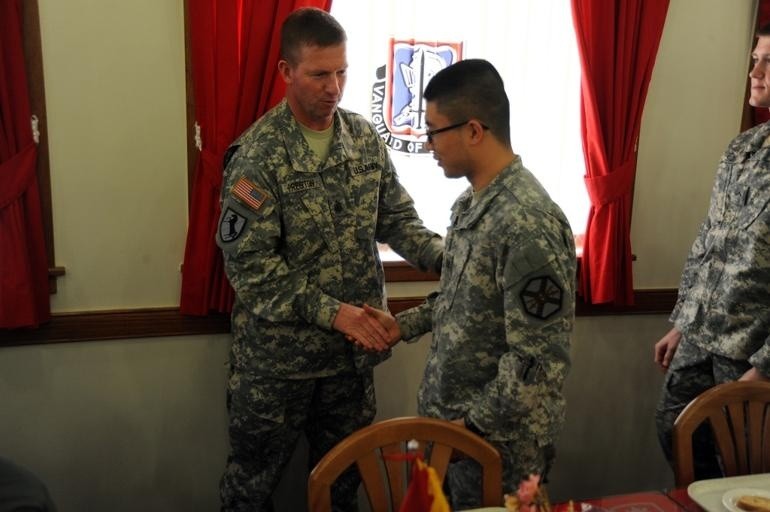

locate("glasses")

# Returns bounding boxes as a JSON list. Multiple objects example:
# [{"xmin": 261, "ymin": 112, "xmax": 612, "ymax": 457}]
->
[{"xmin": 426, "ymin": 120, "xmax": 490, "ymax": 144}]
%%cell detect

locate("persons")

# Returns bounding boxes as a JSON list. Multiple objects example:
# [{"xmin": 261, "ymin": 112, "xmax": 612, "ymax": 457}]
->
[
  {"xmin": 653, "ymin": 25, "xmax": 770, "ymax": 484},
  {"xmin": 215, "ymin": 8, "xmax": 446, "ymax": 512},
  {"xmin": 345, "ymin": 51, "xmax": 576, "ymax": 508}
]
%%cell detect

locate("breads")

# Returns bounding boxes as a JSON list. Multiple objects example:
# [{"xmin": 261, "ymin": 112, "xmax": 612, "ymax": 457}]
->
[{"xmin": 737, "ymin": 495, "xmax": 770, "ymax": 512}]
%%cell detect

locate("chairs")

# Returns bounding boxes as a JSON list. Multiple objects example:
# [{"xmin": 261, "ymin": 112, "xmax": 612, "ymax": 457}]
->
[
  {"xmin": 670, "ymin": 376, "xmax": 770, "ymax": 489},
  {"xmin": 305, "ymin": 415, "xmax": 505, "ymax": 512}
]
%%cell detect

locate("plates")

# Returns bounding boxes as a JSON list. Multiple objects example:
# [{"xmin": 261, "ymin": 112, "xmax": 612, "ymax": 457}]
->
[{"xmin": 720, "ymin": 488, "xmax": 770, "ymax": 511}]
